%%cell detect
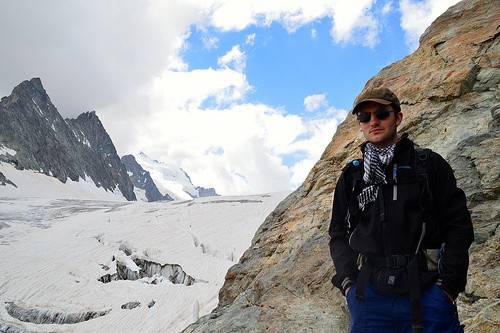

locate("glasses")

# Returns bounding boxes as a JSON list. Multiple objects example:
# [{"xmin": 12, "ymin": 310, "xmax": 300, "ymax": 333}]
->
[{"xmin": 356, "ymin": 108, "xmax": 397, "ymax": 122}]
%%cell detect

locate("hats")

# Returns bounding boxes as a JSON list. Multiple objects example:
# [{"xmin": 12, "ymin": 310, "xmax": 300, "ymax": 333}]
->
[{"xmin": 351, "ymin": 86, "xmax": 401, "ymax": 115}]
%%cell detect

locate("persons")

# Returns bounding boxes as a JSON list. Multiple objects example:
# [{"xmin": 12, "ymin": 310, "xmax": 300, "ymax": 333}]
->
[{"xmin": 329, "ymin": 87, "xmax": 474, "ymax": 333}]
